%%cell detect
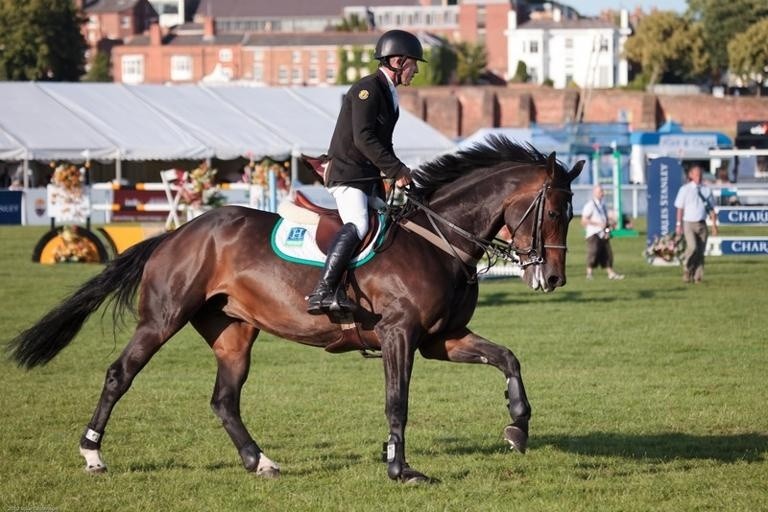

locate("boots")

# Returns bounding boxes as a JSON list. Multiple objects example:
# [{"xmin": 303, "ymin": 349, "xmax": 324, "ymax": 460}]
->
[{"xmin": 306, "ymin": 222, "xmax": 360, "ymax": 314}]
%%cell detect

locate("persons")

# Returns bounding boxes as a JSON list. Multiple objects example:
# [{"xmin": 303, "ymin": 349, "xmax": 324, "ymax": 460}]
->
[
  {"xmin": 305, "ymin": 29, "xmax": 427, "ymax": 315},
  {"xmin": 581, "ymin": 185, "xmax": 624, "ymax": 280},
  {"xmin": 674, "ymin": 164, "xmax": 740, "ymax": 283}
]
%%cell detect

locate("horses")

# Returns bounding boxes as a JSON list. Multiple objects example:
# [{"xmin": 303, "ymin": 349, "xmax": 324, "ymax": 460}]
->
[{"xmin": 3, "ymin": 130, "xmax": 587, "ymax": 487}]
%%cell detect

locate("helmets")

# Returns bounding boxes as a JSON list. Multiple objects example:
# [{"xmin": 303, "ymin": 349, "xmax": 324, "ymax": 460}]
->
[{"xmin": 374, "ymin": 30, "xmax": 427, "ymax": 62}]
